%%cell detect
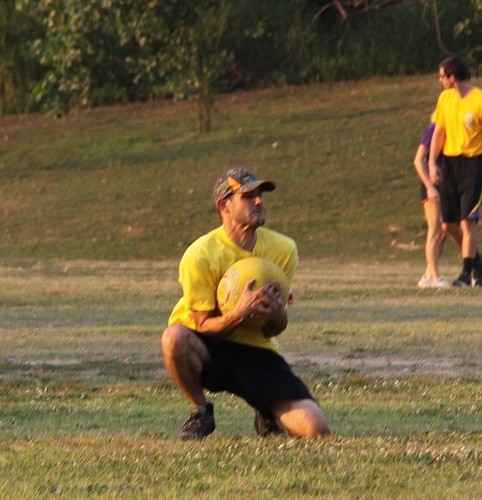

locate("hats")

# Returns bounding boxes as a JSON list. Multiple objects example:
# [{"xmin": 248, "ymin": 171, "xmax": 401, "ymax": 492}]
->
[{"xmin": 213, "ymin": 169, "xmax": 276, "ymax": 206}]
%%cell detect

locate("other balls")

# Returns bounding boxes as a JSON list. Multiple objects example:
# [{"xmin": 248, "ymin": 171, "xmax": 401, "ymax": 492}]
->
[{"xmin": 216, "ymin": 256, "xmax": 290, "ymax": 317}]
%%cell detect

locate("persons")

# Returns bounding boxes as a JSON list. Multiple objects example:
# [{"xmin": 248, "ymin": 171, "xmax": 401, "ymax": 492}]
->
[
  {"xmin": 161, "ymin": 169, "xmax": 332, "ymax": 438},
  {"xmin": 413, "ymin": 114, "xmax": 454, "ymax": 288},
  {"xmin": 428, "ymin": 58, "xmax": 482, "ymax": 286}
]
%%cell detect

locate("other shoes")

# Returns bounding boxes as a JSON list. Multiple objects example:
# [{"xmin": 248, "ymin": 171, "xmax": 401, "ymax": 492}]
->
[
  {"xmin": 179, "ymin": 403, "xmax": 215, "ymax": 441},
  {"xmin": 418, "ymin": 276, "xmax": 452, "ymax": 289},
  {"xmin": 255, "ymin": 410, "xmax": 277, "ymax": 435},
  {"xmin": 453, "ymin": 277, "xmax": 472, "ymax": 286}
]
